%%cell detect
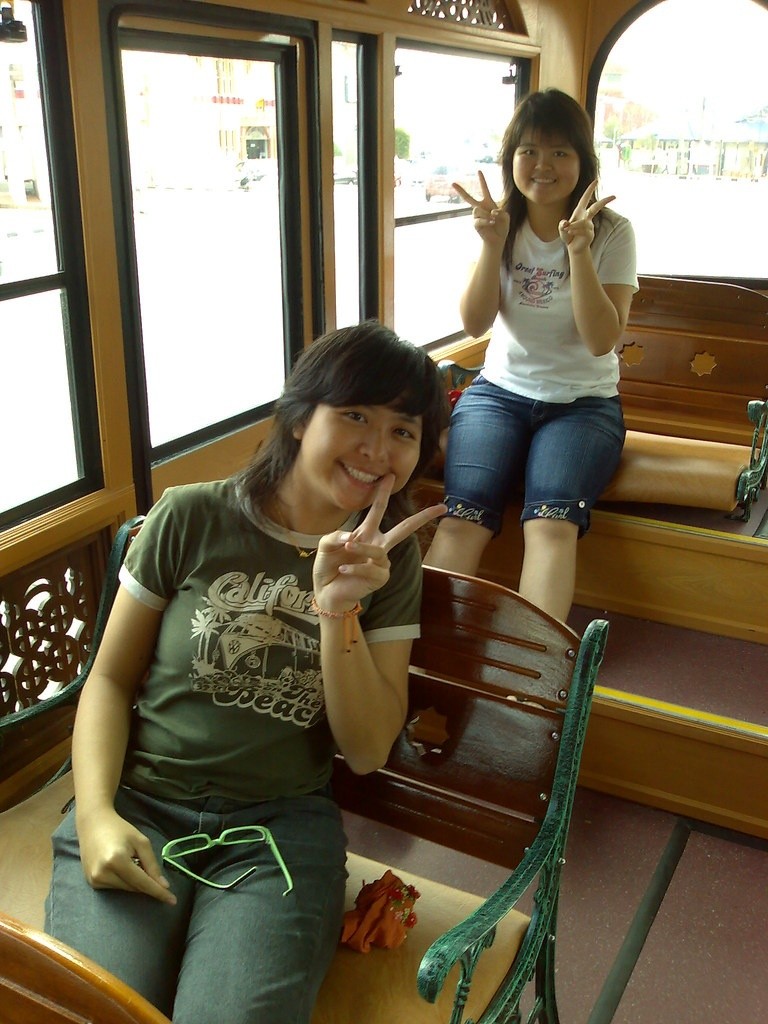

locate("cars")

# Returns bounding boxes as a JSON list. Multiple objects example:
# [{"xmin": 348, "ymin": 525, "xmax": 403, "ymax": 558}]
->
[
  {"xmin": 425, "ymin": 157, "xmax": 491, "ymax": 204},
  {"xmin": 333, "ymin": 155, "xmax": 358, "ymax": 185}
]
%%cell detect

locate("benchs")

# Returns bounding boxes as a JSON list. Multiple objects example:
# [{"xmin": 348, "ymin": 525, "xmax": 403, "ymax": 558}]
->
[
  {"xmin": 0, "ymin": 515, "xmax": 610, "ymax": 1024},
  {"xmin": 436, "ymin": 275, "xmax": 768, "ymax": 524}
]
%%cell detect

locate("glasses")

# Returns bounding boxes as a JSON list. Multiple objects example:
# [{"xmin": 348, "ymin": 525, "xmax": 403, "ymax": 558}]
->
[{"xmin": 160, "ymin": 825, "xmax": 294, "ymax": 897}]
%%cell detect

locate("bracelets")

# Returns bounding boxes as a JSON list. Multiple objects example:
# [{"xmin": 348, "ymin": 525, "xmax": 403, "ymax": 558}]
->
[{"xmin": 311, "ymin": 595, "xmax": 363, "ymax": 655}]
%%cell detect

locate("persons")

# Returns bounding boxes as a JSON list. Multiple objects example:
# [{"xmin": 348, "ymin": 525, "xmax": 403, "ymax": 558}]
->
[
  {"xmin": 44, "ymin": 317, "xmax": 448, "ymax": 1024},
  {"xmin": 421, "ymin": 89, "xmax": 640, "ymax": 625}
]
